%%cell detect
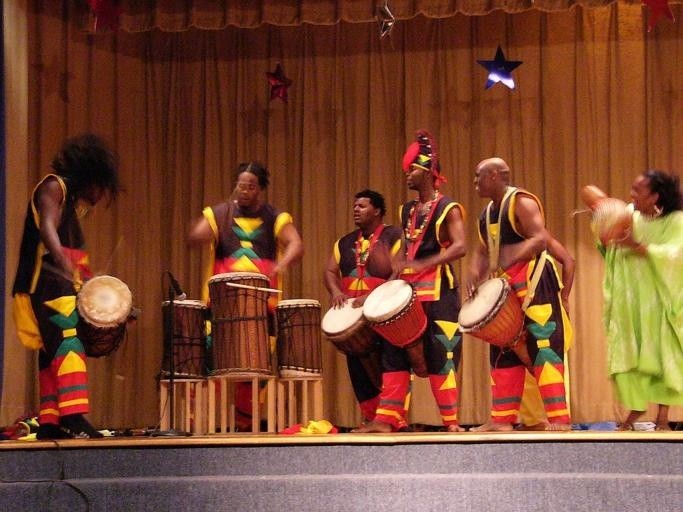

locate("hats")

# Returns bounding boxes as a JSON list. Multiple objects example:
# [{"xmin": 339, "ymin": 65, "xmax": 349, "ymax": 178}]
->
[{"xmin": 408, "ymin": 149, "xmax": 441, "ymax": 176}]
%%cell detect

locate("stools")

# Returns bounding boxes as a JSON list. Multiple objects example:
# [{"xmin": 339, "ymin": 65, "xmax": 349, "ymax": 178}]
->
[{"xmin": 158, "ymin": 374, "xmax": 324, "ymax": 436}]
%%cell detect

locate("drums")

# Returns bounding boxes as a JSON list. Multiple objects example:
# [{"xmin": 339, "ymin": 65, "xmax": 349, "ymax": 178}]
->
[
  {"xmin": 363, "ymin": 283, "xmax": 427, "ymax": 379},
  {"xmin": 321, "ymin": 299, "xmax": 381, "ymax": 389},
  {"xmin": 159, "ymin": 299, "xmax": 205, "ymax": 381},
  {"xmin": 458, "ymin": 279, "xmax": 536, "ymax": 379},
  {"xmin": 208, "ymin": 272, "xmax": 272, "ymax": 380},
  {"xmin": 275, "ymin": 297, "xmax": 322, "ymax": 380},
  {"xmin": 76, "ymin": 274, "xmax": 131, "ymax": 358}
]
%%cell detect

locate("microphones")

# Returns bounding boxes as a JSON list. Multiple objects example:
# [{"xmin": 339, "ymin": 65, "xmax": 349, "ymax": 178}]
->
[{"xmin": 166, "ymin": 270, "xmax": 187, "ymax": 301}]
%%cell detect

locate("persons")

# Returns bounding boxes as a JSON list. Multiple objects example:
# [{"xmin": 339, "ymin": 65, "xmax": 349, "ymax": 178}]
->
[
  {"xmin": 462, "ymin": 157, "xmax": 574, "ymax": 433},
  {"xmin": 580, "ymin": 169, "xmax": 683, "ymax": 430},
  {"xmin": 514, "ymin": 227, "xmax": 574, "ymax": 431},
  {"xmin": 345, "ymin": 127, "xmax": 469, "ymax": 434},
  {"xmin": 321, "ymin": 188, "xmax": 402, "ymax": 433},
  {"xmin": 9, "ymin": 130, "xmax": 125, "ymax": 440},
  {"xmin": 185, "ymin": 161, "xmax": 303, "ymax": 437}
]
